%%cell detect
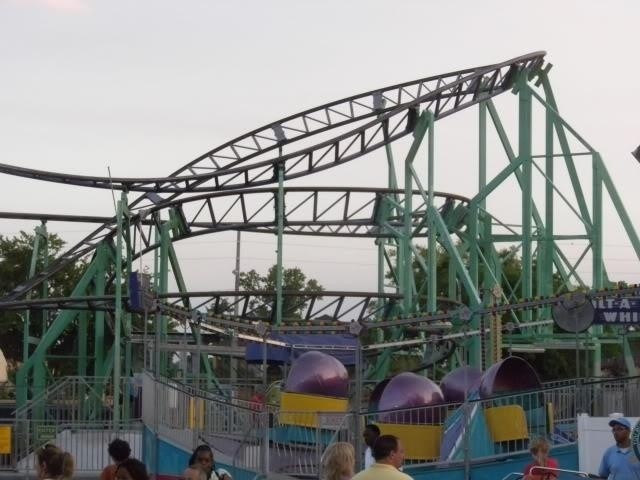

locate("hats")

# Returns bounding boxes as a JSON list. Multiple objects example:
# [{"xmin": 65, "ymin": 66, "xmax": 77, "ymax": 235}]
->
[{"xmin": 608, "ymin": 417, "xmax": 631, "ymax": 430}]
